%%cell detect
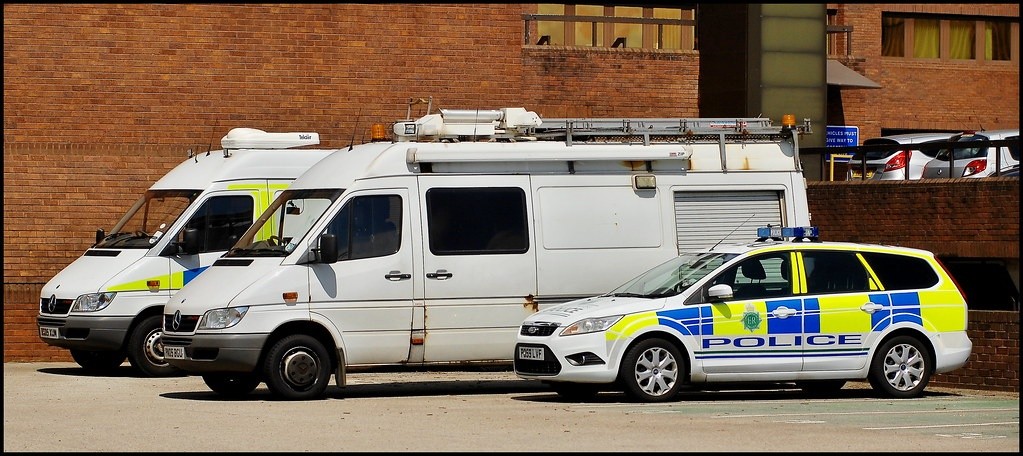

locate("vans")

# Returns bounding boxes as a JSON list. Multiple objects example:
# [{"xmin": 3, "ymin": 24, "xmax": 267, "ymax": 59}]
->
[
  {"xmin": 161, "ymin": 96, "xmax": 815, "ymax": 399},
  {"xmin": 36, "ymin": 126, "xmax": 333, "ymax": 378}
]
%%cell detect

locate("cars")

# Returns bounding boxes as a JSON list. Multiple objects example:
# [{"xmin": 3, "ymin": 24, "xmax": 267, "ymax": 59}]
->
[{"xmin": 513, "ymin": 224, "xmax": 973, "ymax": 403}]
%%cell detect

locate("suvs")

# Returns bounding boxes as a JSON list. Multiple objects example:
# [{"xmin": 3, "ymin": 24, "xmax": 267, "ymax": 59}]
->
[
  {"xmin": 921, "ymin": 130, "xmax": 1020, "ymax": 179},
  {"xmin": 847, "ymin": 133, "xmax": 959, "ymax": 180}
]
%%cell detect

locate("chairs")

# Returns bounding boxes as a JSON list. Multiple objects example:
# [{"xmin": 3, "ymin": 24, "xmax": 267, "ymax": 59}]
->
[
  {"xmin": 777, "ymin": 261, "xmax": 792, "ymax": 297},
  {"xmin": 363, "ymin": 198, "xmax": 399, "ymax": 255},
  {"xmin": 740, "ymin": 259, "xmax": 767, "ymax": 298}
]
